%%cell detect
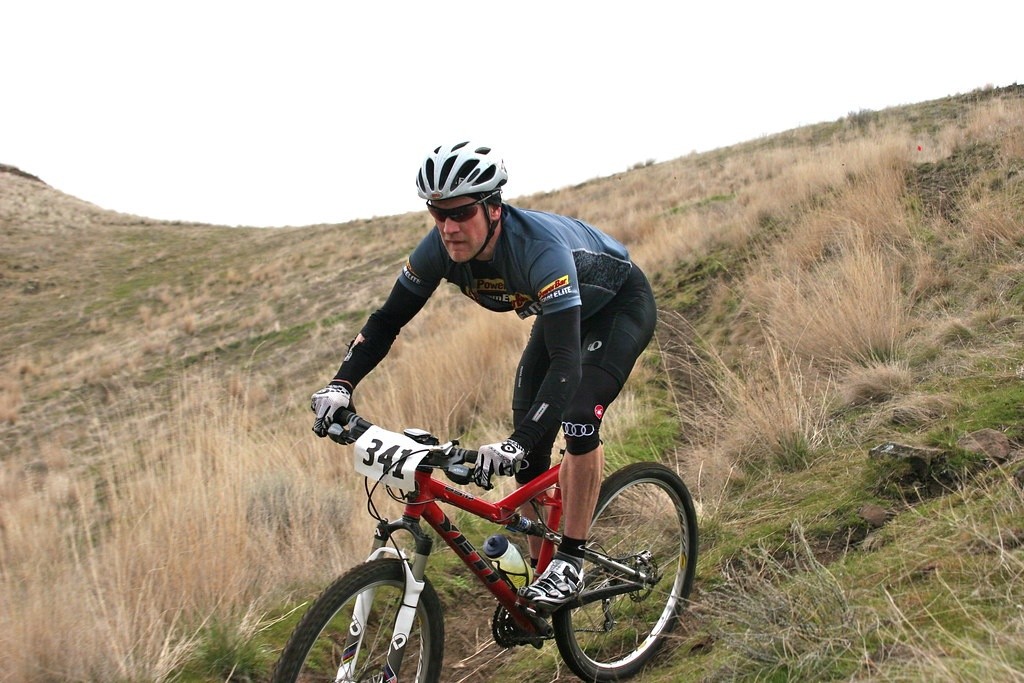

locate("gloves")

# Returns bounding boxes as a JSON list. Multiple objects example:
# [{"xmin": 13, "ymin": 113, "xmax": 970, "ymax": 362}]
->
[
  {"xmin": 472, "ymin": 439, "xmax": 531, "ymax": 492},
  {"xmin": 311, "ymin": 384, "xmax": 358, "ymax": 438}
]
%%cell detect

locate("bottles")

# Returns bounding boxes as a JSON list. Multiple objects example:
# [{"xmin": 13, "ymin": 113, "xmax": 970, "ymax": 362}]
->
[{"xmin": 483, "ymin": 535, "xmax": 533, "ymax": 589}]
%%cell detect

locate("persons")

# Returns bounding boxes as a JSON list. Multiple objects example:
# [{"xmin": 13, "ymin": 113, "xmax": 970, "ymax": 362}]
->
[{"xmin": 310, "ymin": 140, "xmax": 656, "ymax": 604}]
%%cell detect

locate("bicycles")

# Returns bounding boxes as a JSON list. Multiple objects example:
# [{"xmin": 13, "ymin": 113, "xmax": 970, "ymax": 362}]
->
[{"xmin": 268, "ymin": 396, "xmax": 699, "ymax": 683}]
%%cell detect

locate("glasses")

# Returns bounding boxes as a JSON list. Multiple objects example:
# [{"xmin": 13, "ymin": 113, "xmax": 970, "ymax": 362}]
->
[{"xmin": 423, "ymin": 190, "xmax": 500, "ymax": 224}]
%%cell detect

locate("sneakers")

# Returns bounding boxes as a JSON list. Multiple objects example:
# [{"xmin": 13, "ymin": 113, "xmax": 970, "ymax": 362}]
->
[{"xmin": 516, "ymin": 558, "xmax": 586, "ymax": 605}]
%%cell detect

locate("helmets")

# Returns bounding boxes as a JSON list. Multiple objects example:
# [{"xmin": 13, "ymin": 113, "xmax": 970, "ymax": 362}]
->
[{"xmin": 415, "ymin": 139, "xmax": 507, "ymax": 201}]
